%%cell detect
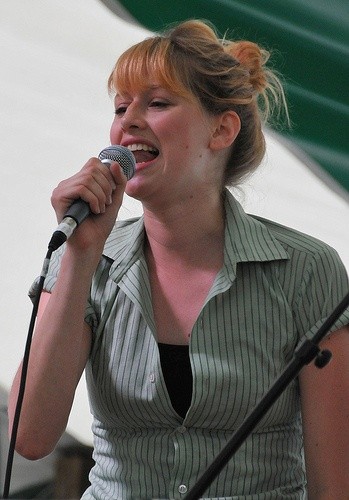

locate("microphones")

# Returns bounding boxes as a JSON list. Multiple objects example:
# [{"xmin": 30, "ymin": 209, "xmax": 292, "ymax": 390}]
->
[{"xmin": 48, "ymin": 145, "xmax": 136, "ymax": 252}]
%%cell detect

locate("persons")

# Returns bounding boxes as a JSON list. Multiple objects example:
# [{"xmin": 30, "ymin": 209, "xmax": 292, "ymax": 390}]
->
[{"xmin": 7, "ymin": 19, "xmax": 349, "ymax": 500}]
